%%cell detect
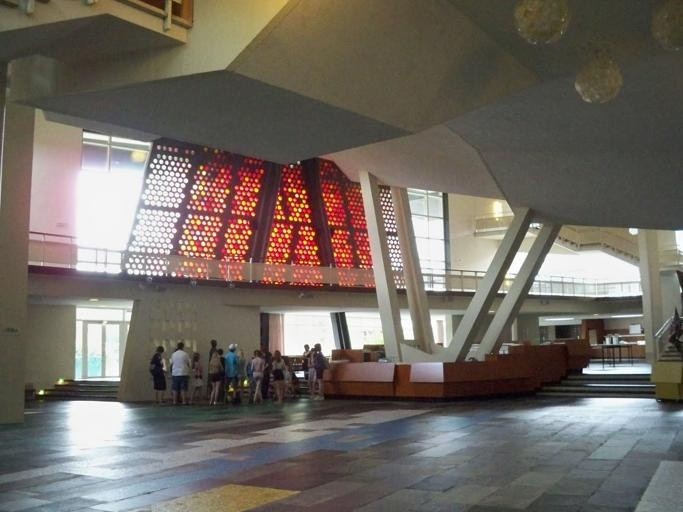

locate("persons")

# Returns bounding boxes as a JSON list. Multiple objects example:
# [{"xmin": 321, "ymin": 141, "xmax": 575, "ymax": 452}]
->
[{"xmin": 149, "ymin": 340, "xmax": 329, "ymax": 407}]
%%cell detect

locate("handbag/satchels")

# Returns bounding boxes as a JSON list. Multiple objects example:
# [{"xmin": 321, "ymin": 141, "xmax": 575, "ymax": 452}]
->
[
  {"xmin": 319, "ymin": 358, "xmax": 330, "ymax": 369},
  {"xmin": 292, "ymin": 375, "xmax": 298, "ymax": 384}
]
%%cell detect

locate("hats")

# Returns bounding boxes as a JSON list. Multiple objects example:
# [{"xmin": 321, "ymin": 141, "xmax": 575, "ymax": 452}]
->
[{"xmin": 229, "ymin": 344, "xmax": 238, "ymax": 350}]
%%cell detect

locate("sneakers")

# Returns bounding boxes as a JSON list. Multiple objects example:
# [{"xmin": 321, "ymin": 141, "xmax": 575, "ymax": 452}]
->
[{"xmin": 311, "ymin": 395, "xmax": 325, "ymax": 401}]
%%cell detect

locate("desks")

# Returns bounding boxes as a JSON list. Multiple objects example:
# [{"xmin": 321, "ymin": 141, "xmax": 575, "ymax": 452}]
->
[{"xmin": 601, "ymin": 344, "xmax": 633, "ymax": 368}]
